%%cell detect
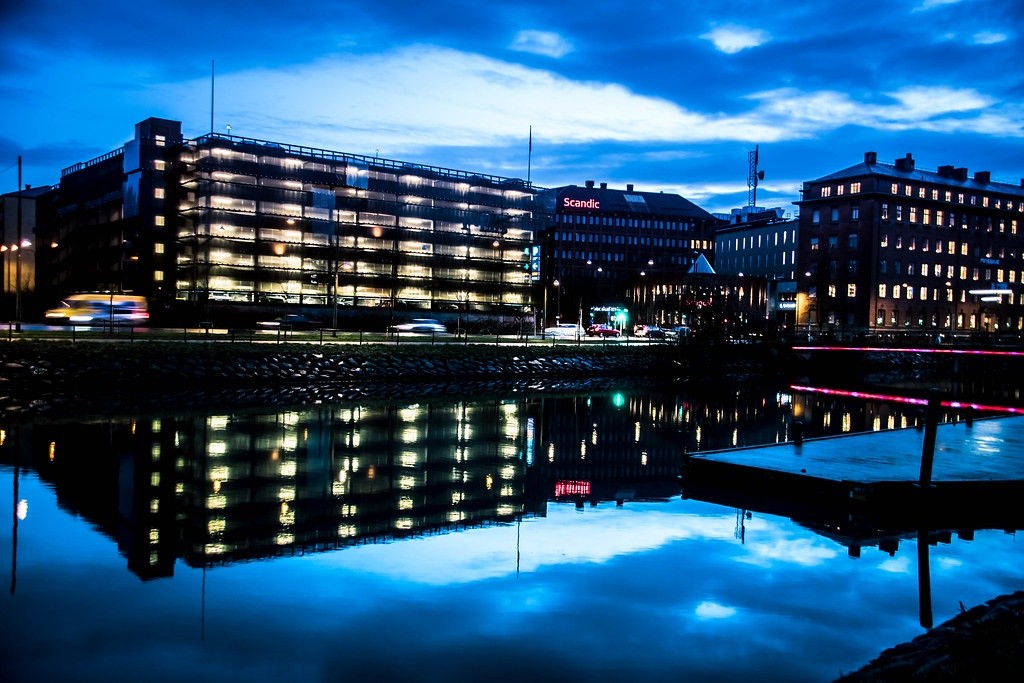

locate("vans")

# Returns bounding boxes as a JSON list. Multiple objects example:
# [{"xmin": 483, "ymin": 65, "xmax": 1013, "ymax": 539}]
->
[{"xmin": 45, "ymin": 294, "xmax": 150, "ymax": 325}]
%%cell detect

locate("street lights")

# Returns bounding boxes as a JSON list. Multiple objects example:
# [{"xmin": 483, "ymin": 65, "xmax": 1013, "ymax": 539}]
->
[
  {"xmin": 797, "ymin": 270, "xmax": 811, "ymax": 331},
  {"xmin": 543, "ymin": 274, "xmax": 560, "ymax": 340},
  {"xmin": 554, "ymin": 278, "xmax": 560, "ymax": 327},
  {"xmin": 841, "ymin": 299, "xmax": 857, "ymax": 342}
]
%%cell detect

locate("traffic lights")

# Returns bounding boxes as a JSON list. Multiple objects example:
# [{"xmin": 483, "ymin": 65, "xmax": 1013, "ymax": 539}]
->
[{"xmin": 522, "ymin": 263, "xmax": 530, "ymax": 271}]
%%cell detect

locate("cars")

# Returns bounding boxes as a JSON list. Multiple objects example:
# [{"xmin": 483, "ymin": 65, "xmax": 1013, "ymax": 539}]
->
[
  {"xmin": 647, "ymin": 327, "xmax": 669, "ymax": 338},
  {"xmin": 410, "ymin": 319, "xmax": 447, "ymax": 332},
  {"xmin": 589, "ymin": 324, "xmax": 622, "ymax": 338},
  {"xmin": 276, "ymin": 314, "xmax": 323, "ymax": 330},
  {"xmin": 91, "ymin": 306, "xmax": 149, "ymax": 326},
  {"xmin": 666, "ymin": 326, "xmax": 692, "ymax": 338},
  {"xmin": 545, "ymin": 324, "xmax": 586, "ymax": 337}
]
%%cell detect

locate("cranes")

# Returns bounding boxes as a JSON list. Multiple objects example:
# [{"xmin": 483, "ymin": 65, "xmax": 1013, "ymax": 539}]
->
[{"xmin": 747, "ymin": 145, "xmax": 759, "ymax": 206}]
